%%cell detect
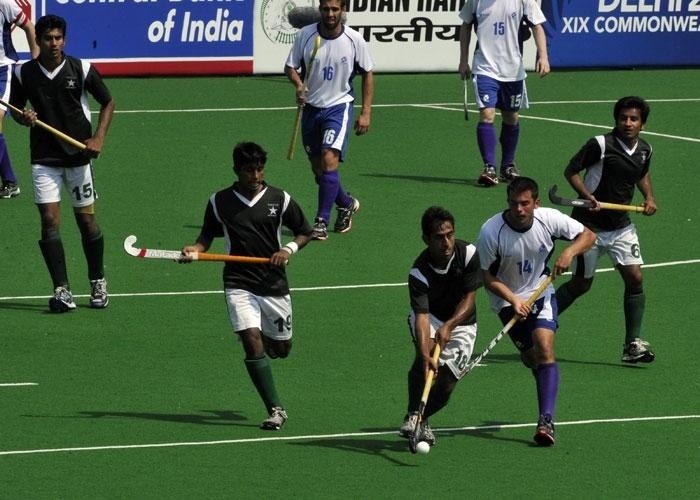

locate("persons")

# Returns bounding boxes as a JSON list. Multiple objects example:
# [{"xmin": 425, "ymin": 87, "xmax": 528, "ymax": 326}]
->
[
  {"xmin": 1, "ymin": 0, "xmax": 40, "ymax": 199},
  {"xmin": 400, "ymin": 205, "xmax": 485, "ymax": 446},
  {"xmin": 10, "ymin": 16, "xmax": 114, "ymax": 312},
  {"xmin": 554, "ymin": 96, "xmax": 657, "ymax": 364},
  {"xmin": 475, "ymin": 178, "xmax": 598, "ymax": 446},
  {"xmin": 175, "ymin": 141, "xmax": 313, "ymax": 429},
  {"xmin": 284, "ymin": 0, "xmax": 374, "ymax": 240},
  {"xmin": 457, "ymin": 0, "xmax": 551, "ymax": 185}
]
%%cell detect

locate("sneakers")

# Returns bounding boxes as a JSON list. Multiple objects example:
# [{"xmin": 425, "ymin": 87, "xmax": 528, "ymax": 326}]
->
[
  {"xmin": 48, "ymin": 287, "xmax": 77, "ymax": 312},
  {"xmin": 311, "ymin": 216, "xmax": 328, "ymax": 240},
  {"xmin": 621, "ymin": 337, "xmax": 655, "ymax": 363},
  {"xmin": 498, "ymin": 164, "xmax": 521, "ymax": 183},
  {"xmin": 415, "ymin": 417, "xmax": 436, "ymax": 446},
  {"xmin": 400, "ymin": 411, "xmax": 419, "ymax": 438},
  {"xmin": 260, "ymin": 407, "xmax": 288, "ymax": 430},
  {"xmin": 334, "ymin": 192, "xmax": 360, "ymax": 233},
  {"xmin": 478, "ymin": 163, "xmax": 498, "ymax": 186},
  {"xmin": 534, "ymin": 413, "xmax": 555, "ymax": 447},
  {"xmin": 0, "ymin": 182, "xmax": 20, "ymax": 199},
  {"xmin": 89, "ymin": 277, "xmax": 109, "ymax": 308}
]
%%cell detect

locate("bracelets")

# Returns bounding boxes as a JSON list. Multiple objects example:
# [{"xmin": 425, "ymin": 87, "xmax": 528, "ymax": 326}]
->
[{"xmin": 281, "ymin": 241, "xmax": 299, "ymax": 256}]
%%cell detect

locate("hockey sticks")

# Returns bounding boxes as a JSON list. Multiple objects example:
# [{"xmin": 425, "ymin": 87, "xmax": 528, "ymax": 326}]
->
[
  {"xmin": 460, "ymin": 275, "xmax": 552, "ymax": 379},
  {"xmin": 548, "ymin": 184, "xmax": 646, "ymax": 213},
  {"xmin": 287, "ymin": 36, "xmax": 320, "ymax": 160},
  {"xmin": 408, "ymin": 343, "xmax": 440, "ymax": 453},
  {"xmin": 464, "ymin": 73, "xmax": 468, "ymax": 120},
  {"xmin": 124, "ymin": 235, "xmax": 290, "ymax": 266}
]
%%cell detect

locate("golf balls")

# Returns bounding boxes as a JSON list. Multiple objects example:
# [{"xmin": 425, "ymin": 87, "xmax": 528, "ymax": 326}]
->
[{"xmin": 416, "ymin": 440, "xmax": 430, "ymax": 454}]
